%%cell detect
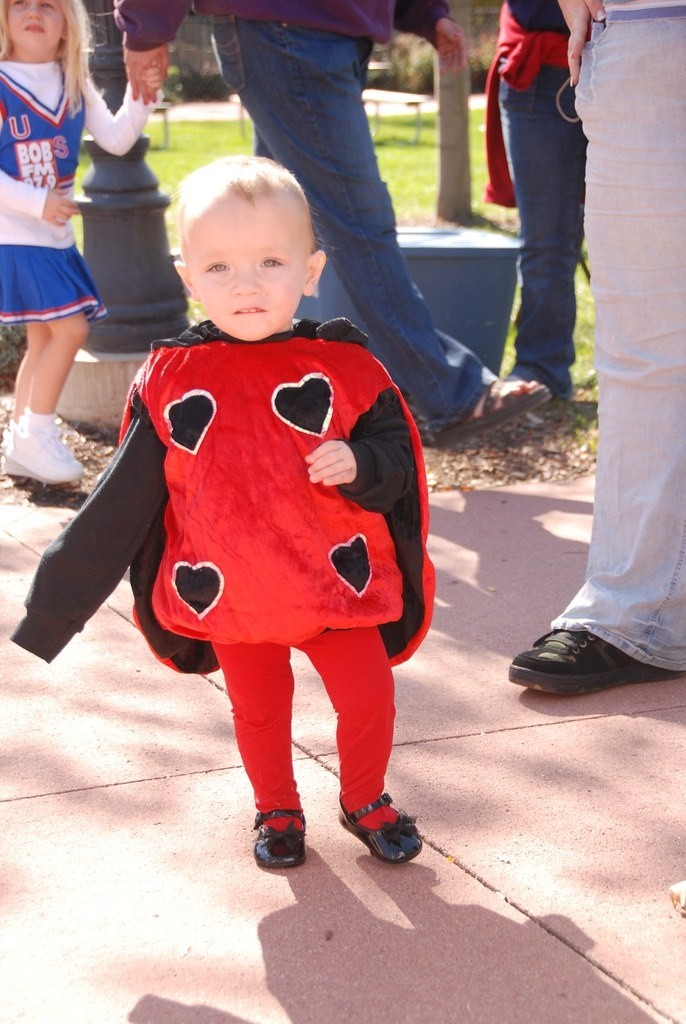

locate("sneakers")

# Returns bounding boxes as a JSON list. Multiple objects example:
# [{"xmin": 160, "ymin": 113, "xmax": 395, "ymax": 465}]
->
[
  {"xmin": 2, "ymin": 414, "xmax": 83, "ymax": 485},
  {"xmin": 507, "ymin": 629, "xmax": 686, "ymax": 694}
]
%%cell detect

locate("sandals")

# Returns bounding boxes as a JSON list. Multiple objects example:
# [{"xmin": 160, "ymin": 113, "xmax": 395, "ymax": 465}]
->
[{"xmin": 436, "ymin": 377, "xmax": 551, "ymax": 444}]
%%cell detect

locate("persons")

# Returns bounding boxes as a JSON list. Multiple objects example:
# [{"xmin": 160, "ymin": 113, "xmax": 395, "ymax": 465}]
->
[
  {"xmin": 508, "ymin": 0, "xmax": 686, "ymax": 695},
  {"xmin": 114, "ymin": 0, "xmax": 551, "ymax": 448},
  {"xmin": 482, "ymin": 0, "xmax": 590, "ymax": 404},
  {"xmin": 6, "ymin": 156, "xmax": 436, "ymax": 868},
  {"xmin": 0, "ymin": 0, "xmax": 165, "ymax": 484}
]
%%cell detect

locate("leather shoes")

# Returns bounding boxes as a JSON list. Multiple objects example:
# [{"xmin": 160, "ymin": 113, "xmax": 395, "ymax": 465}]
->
[
  {"xmin": 252, "ymin": 809, "xmax": 306, "ymax": 868},
  {"xmin": 338, "ymin": 790, "xmax": 422, "ymax": 864}
]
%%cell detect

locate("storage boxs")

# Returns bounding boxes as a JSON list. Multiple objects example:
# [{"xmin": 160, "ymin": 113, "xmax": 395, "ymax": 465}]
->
[{"xmin": 293, "ymin": 225, "xmax": 522, "ymax": 391}]
